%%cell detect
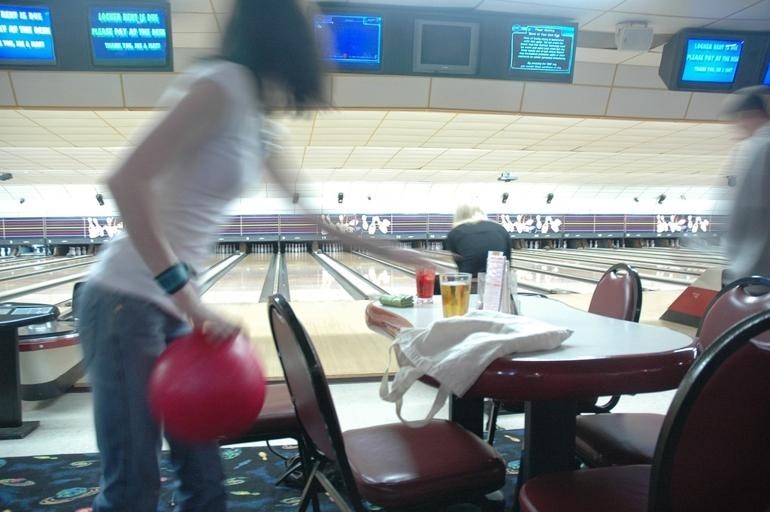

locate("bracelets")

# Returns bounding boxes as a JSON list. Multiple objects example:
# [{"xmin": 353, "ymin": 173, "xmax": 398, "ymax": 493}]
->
[{"xmin": 154, "ymin": 259, "xmax": 192, "ymax": 295}]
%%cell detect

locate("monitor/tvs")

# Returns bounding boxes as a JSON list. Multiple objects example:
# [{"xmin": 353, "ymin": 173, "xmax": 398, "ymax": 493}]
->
[
  {"xmin": 86, "ymin": 0, "xmax": 175, "ymax": 72},
  {"xmin": 412, "ymin": 21, "xmax": 479, "ymax": 75},
  {"xmin": 0, "ymin": 0, "xmax": 62, "ymax": 68},
  {"xmin": 755, "ymin": 43, "xmax": 770, "ymax": 86},
  {"xmin": 311, "ymin": 6, "xmax": 390, "ymax": 74},
  {"xmin": 503, "ymin": 17, "xmax": 579, "ymax": 82},
  {"xmin": 658, "ymin": 28, "xmax": 746, "ymax": 93}
]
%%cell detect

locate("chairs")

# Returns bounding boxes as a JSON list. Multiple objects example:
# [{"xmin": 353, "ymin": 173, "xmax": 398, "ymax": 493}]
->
[
  {"xmin": 576, "ymin": 276, "xmax": 770, "ymax": 469},
  {"xmin": 485, "ymin": 263, "xmax": 643, "ymax": 446},
  {"xmin": 215, "ymin": 380, "xmax": 322, "ymax": 511},
  {"xmin": 267, "ymin": 293, "xmax": 507, "ymax": 511},
  {"xmin": 518, "ymin": 310, "xmax": 770, "ymax": 512}
]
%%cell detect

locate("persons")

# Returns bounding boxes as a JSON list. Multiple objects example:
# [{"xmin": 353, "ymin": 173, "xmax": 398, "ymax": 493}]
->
[
  {"xmin": 714, "ymin": 84, "xmax": 769, "ymax": 295},
  {"xmin": 79, "ymin": 1, "xmax": 432, "ymax": 511},
  {"xmin": 441, "ymin": 204, "xmax": 512, "ymax": 278}
]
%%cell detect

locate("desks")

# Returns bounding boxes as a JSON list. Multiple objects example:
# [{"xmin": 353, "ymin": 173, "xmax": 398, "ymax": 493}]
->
[
  {"xmin": 0, "ymin": 303, "xmax": 59, "ymax": 439},
  {"xmin": 365, "ymin": 295, "xmax": 703, "ymax": 500}
]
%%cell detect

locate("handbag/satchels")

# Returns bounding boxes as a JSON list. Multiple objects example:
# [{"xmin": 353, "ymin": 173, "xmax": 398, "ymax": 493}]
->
[{"xmin": 375, "ymin": 307, "xmax": 577, "ymax": 431}]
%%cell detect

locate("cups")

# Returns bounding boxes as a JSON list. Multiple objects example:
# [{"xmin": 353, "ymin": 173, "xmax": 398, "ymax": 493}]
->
[
  {"xmin": 437, "ymin": 271, "xmax": 470, "ymax": 321},
  {"xmin": 477, "ymin": 246, "xmax": 520, "ymax": 313},
  {"xmin": 415, "ymin": 266, "xmax": 435, "ymax": 308}
]
